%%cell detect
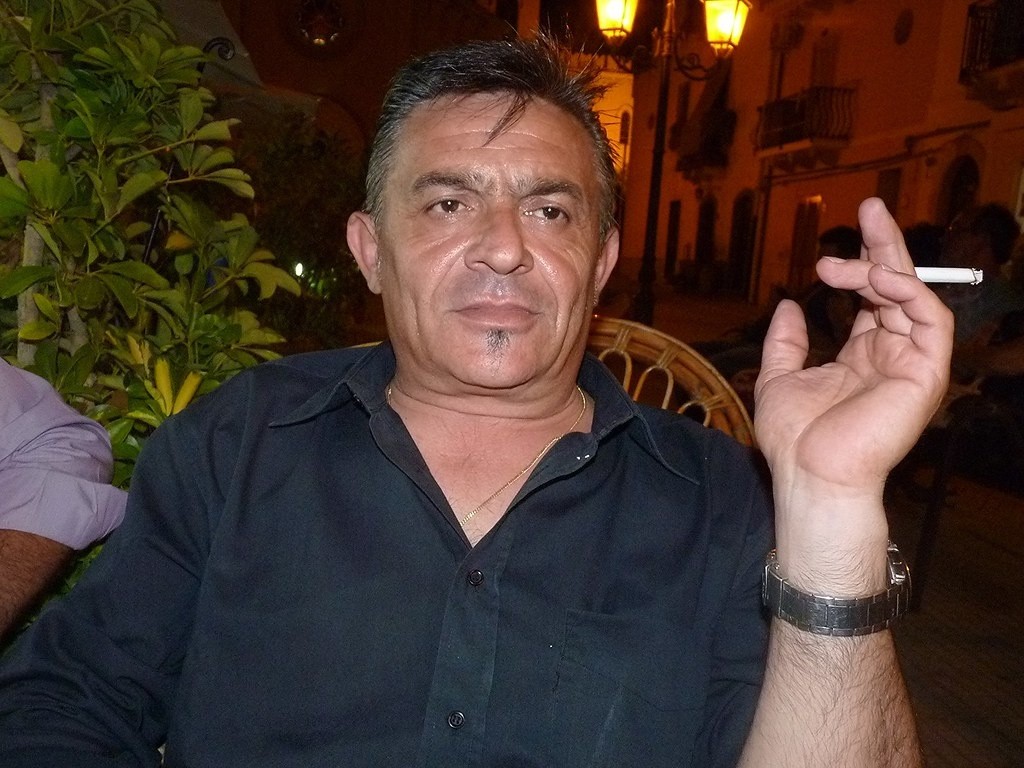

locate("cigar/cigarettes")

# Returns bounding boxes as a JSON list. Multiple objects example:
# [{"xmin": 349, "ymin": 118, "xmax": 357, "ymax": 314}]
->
[{"xmin": 914, "ymin": 267, "xmax": 983, "ymax": 283}]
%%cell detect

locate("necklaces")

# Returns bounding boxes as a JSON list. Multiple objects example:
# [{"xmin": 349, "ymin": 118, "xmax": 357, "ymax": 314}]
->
[{"xmin": 383, "ymin": 380, "xmax": 588, "ymax": 528}]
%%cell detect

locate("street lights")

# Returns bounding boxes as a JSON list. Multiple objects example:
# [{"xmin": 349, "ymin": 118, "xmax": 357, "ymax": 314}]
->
[{"xmin": 596, "ymin": 0, "xmax": 754, "ymax": 331}]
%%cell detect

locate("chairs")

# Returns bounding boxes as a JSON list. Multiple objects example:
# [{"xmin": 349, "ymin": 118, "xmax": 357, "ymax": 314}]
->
[
  {"xmin": 882, "ymin": 269, "xmax": 1024, "ymax": 615},
  {"xmin": 349, "ymin": 315, "xmax": 759, "ymax": 454}
]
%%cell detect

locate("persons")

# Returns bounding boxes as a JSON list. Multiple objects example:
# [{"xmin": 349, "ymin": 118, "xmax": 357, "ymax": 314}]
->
[
  {"xmin": 673, "ymin": 197, "xmax": 1024, "ymax": 507},
  {"xmin": 0, "ymin": 357, "xmax": 129, "ymax": 640},
  {"xmin": 1, "ymin": 38, "xmax": 955, "ymax": 768}
]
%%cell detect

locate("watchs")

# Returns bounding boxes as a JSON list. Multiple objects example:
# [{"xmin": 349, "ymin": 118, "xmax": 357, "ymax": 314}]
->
[{"xmin": 762, "ymin": 540, "xmax": 912, "ymax": 638}]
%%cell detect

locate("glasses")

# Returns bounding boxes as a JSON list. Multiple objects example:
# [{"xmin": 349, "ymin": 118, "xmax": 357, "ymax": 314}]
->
[{"xmin": 944, "ymin": 222, "xmax": 976, "ymax": 240}]
